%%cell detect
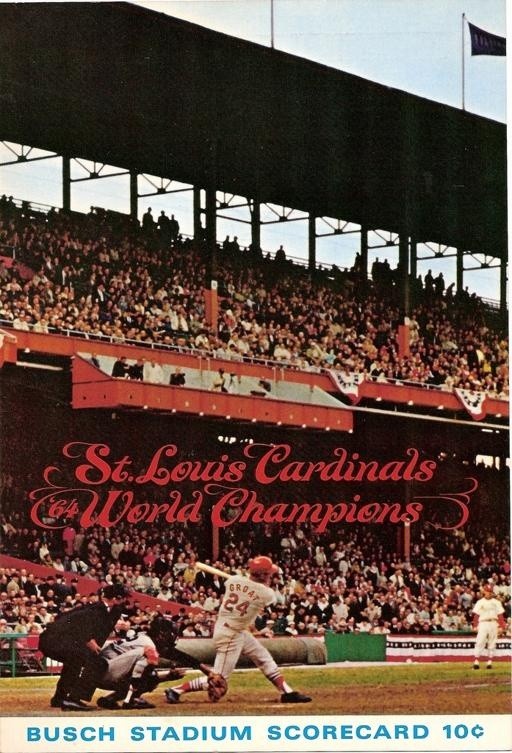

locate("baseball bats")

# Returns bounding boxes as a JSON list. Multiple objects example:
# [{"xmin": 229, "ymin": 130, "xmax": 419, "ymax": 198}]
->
[{"xmin": 196, "ymin": 562, "xmax": 233, "ymax": 579}]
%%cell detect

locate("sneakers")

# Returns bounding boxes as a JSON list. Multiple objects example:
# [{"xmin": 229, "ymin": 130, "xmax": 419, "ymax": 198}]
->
[
  {"xmin": 165, "ymin": 688, "xmax": 180, "ymax": 703},
  {"xmin": 281, "ymin": 691, "xmax": 311, "ymax": 703},
  {"xmin": 51, "ymin": 694, "xmax": 156, "ymax": 710}
]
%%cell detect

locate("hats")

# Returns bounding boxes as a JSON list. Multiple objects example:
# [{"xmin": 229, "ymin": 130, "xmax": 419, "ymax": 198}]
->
[{"xmin": 102, "ymin": 584, "xmax": 131, "ymax": 597}]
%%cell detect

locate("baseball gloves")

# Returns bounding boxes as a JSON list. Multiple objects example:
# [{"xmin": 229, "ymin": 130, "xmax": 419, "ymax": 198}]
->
[{"xmin": 207, "ymin": 673, "xmax": 228, "ymax": 702}]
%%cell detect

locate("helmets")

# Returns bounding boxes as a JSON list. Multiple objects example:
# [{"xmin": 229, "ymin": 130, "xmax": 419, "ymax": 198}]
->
[
  {"xmin": 250, "ymin": 556, "xmax": 277, "ymax": 572},
  {"xmin": 150, "ymin": 621, "xmax": 175, "ymax": 655}
]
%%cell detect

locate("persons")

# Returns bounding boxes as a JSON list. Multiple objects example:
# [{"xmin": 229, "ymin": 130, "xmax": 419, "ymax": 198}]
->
[
  {"xmin": 38, "ymin": 580, "xmax": 131, "ymax": 713},
  {"xmin": 92, "ymin": 616, "xmax": 214, "ymax": 711},
  {"xmin": 0, "ymin": 193, "xmax": 509, "ymax": 669},
  {"xmin": 163, "ymin": 555, "xmax": 313, "ymax": 704},
  {"xmin": 467, "ymin": 21, "xmax": 502, "ymax": 54}
]
